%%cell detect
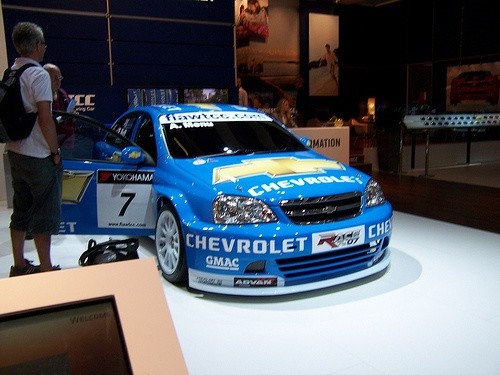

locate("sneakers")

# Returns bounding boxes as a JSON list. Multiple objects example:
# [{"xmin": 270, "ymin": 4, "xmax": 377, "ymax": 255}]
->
[
  {"xmin": 9, "ymin": 259, "xmax": 41, "ymax": 277},
  {"xmin": 51, "ymin": 265, "xmax": 62, "ymax": 270}
]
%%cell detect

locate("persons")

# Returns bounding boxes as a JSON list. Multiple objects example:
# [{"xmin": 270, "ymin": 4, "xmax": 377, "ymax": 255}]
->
[
  {"xmin": 4, "ymin": 22, "xmax": 75, "ymax": 277},
  {"xmin": 318, "ymin": 44, "xmax": 338, "ymax": 87},
  {"xmin": 237, "ymin": 79, "xmax": 248, "ymax": 106},
  {"xmin": 235, "ymin": 0, "xmax": 270, "ymax": 39},
  {"xmin": 271, "ymin": 98, "xmax": 298, "ymax": 128}
]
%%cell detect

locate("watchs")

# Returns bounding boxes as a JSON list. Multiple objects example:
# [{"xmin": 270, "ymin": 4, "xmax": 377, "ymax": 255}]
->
[{"xmin": 51, "ymin": 148, "xmax": 61, "ymax": 155}]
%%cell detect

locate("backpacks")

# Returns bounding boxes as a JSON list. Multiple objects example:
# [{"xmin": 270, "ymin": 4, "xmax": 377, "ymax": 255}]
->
[
  {"xmin": 0, "ymin": 62, "xmax": 41, "ymax": 143},
  {"xmin": 79, "ymin": 238, "xmax": 139, "ymax": 266}
]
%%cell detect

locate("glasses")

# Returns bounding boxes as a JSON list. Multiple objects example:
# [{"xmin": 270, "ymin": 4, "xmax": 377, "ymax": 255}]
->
[
  {"xmin": 42, "ymin": 41, "xmax": 48, "ymax": 48},
  {"xmin": 51, "ymin": 74, "xmax": 63, "ymax": 81}
]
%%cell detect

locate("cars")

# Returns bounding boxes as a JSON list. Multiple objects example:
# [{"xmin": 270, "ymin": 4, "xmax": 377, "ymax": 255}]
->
[{"xmin": 51, "ymin": 101, "xmax": 393, "ymax": 297}]
